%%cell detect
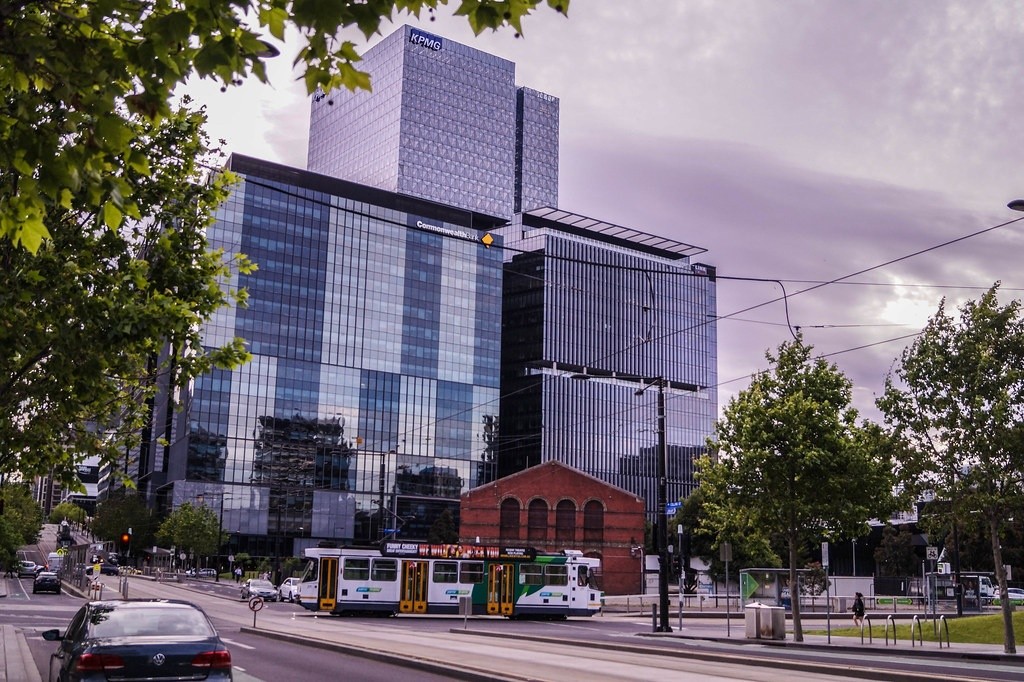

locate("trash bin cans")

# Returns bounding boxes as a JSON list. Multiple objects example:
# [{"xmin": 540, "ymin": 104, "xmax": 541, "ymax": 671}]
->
[{"xmin": 744, "ymin": 601, "xmax": 787, "ymax": 640}]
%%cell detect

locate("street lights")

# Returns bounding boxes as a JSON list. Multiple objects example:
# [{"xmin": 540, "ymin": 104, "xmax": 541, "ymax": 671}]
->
[
  {"xmin": 196, "ymin": 492, "xmax": 224, "ymax": 582},
  {"xmin": 569, "ymin": 373, "xmax": 676, "ymax": 633}
]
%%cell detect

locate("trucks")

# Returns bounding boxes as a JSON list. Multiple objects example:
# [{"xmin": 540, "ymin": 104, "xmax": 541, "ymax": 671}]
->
[{"xmin": 906, "ymin": 574, "xmax": 996, "ymax": 607}]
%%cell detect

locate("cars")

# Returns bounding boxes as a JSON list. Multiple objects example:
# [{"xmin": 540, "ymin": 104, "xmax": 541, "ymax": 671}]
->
[
  {"xmin": 185, "ymin": 568, "xmax": 203, "ymax": 575},
  {"xmin": 86, "ymin": 561, "xmax": 119, "ymax": 576},
  {"xmin": 17, "ymin": 561, "xmax": 38, "ymax": 578},
  {"xmin": 36, "ymin": 519, "xmax": 87, "ymax": 579},
  {"xmin": 193, "ymin": 568, "xmax": 216, "ymax": 576},
  {"xmin": 33, "ymin": 571, "xmax": 61, "ymax": 595},
  {"xmin": 41, "ymin": 597, "xmax": 233, "ymax": 682},
  {"xmin": 278, "ymin": 577, "xmax": 302, "ymax": 603},
  {"xmin": 116, "ymin": 566, "xmax": 143, "ymax": 576},
  {"xmin": 993, "ymin": 587, "xmax": 1023, "ymax": 606},
  {"xmin": 240, "ymin": 579, "xmax": 278, "ymax": 602}
]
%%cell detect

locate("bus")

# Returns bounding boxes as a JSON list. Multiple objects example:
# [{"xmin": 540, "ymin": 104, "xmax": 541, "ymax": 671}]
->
[{"xmin": 295, "ymin": 541, "xmax": 604, "ymax": 622}]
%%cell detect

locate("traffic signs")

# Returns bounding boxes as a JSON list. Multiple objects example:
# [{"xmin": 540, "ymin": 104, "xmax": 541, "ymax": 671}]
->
[{"xmin": 666, "ymin": 501, "xmax": 682, "ymax": 515}]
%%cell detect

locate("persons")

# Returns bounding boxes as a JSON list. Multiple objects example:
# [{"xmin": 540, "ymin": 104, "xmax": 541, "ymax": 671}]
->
[
  {"xmin": 262, "ymin": 571, "xmax": 270, "ymax": 580},
  {"xmin": 457, "ymin": 545, "xmax": 470, "ymax": 558},
  {"xmin": 235, "ymin": 566, "xmax": 242, "ymax": 583},
  {"xmin": 3, "ymin": 570, "xmax": 14, "ymax": 579},
  {"xmin": 449, "ymin": 547, "xmax": 456, "ymax": 558},
  {"xmin": 852, "ymin": 592, "xmax": 865, "ymax": 626}
]
